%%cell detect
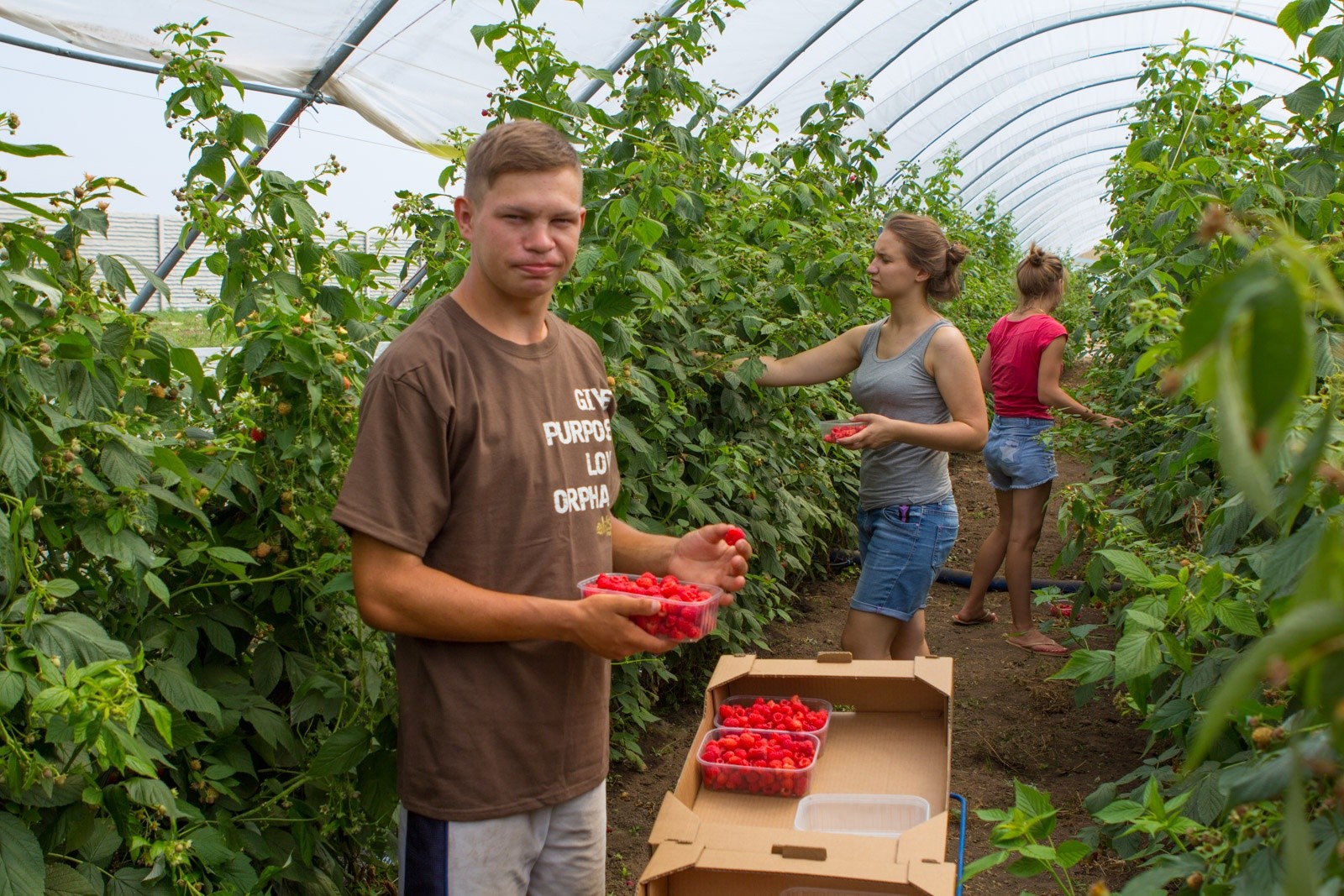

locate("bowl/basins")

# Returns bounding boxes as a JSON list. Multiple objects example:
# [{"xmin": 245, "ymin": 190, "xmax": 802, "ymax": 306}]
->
[
  {"xmin": 577, "ymin": 573, "xmax": 722, "ymax": 642},
  {"xmin": 793, "ymin": 793, "xmax": 930, "ymax": 839},
  {"xmin": 696, "ymin": 692, "xmax": 833, "ymax": 799},
  {"xmin": 818, "ymin": 419, "xmax": 868, "ymax": 443}
]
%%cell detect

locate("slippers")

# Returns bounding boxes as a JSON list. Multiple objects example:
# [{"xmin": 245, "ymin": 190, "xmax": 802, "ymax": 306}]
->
[
  {"xmin": 952, "ymin": 609, "xmax": 998, "ymax": 624},
  {"xmin": 1006, "ymin": 638, "xmax": 1070, "ymax": 657}
]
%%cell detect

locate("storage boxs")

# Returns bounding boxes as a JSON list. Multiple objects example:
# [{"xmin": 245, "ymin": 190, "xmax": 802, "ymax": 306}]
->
[
  {"xmin": 818, "ymin": 418, "xmax": 869, "ymax": 446},
  {"xmin": 1046, "ymin": 598, "xmax": 1083, "ymax": 621},
  {"xmin": 713, "ymin": 693, "xmax": 834, "ymax": 760},
  {"xmin": 574, "ymin": 570, "xmax": 724, "ymax": 646},
  {"xmin": 632, "ymin": 648, "xmax": 962, "ymax": 896},
  {"xmin": 793, "ymin": 790, "xmax": 933, "ymax": 837},
  {"xmin": 694, "ymin": 727, "xmax": 823, "ymax": 799}
]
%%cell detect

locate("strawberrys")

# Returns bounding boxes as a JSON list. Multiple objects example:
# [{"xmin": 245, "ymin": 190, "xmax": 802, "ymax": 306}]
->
[{"xmin": 0, "ymin": 0, "xmax": 1344, "ymax": 895}]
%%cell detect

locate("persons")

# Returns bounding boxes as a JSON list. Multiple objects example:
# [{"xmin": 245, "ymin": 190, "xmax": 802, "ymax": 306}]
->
[
  {"xmin": 332, "ymin": 119, "xmax": 751, "ymax": 896},
  {"xmin": 691, "ymin": 212, "xmax": 989, "ymax": 660},
  {"xmin": 951, "ymin": 241, "xmax": 1133, "ymax": 658}
]
